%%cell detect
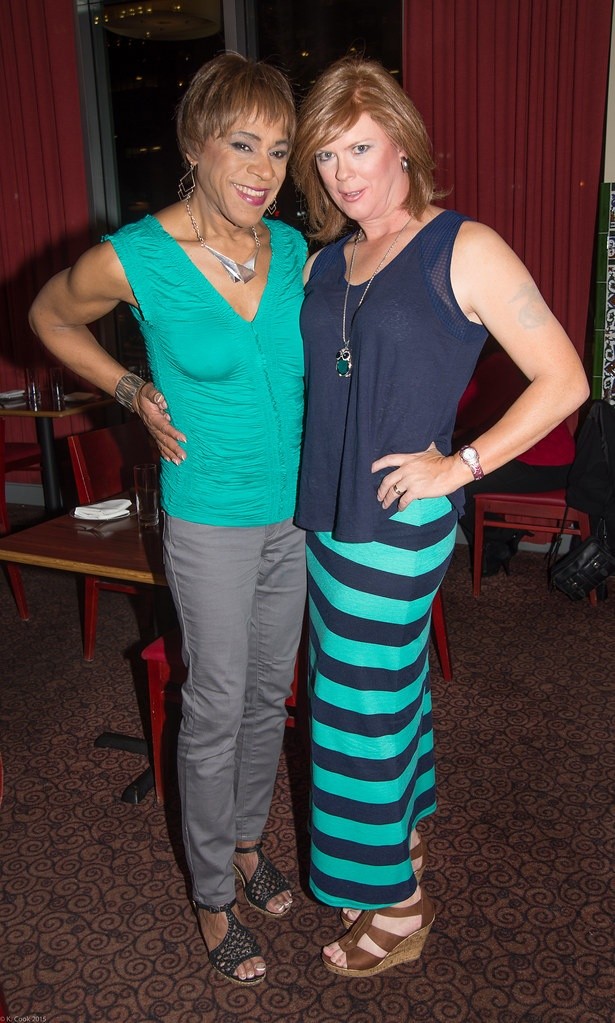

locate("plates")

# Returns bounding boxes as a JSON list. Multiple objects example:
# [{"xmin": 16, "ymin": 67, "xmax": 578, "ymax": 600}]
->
[
  {"xmin": 69, "ymin": 511, "xmax": 131, "ymax": 521},
  {"xmin": 64, "ymin": 391, "xmax": 93, "ymax": 402}
]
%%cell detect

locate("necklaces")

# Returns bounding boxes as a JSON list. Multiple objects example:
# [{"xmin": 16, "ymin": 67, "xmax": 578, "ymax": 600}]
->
[
  {"xmin": 335, "ymin": 212, "xmax": 416, "ymax": 377},
  {"xmin": 186, "ymin": 187, "xmax": 262, "ymax": 284}
]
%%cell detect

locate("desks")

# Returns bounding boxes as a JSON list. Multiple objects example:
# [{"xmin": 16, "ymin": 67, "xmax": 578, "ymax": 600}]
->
[{"xmin": 0, "ymin": 391, "xmax": 120, "ymax": 534}]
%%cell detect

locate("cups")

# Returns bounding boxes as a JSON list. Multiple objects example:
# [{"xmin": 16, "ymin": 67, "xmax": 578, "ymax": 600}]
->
[
  {"xmin": 49, "ymin": 366, "xmax": 65, "ymax": 407},
  {"xmin": 26, "ymin": 368, "xmax": 41, "ymax": 403},
  {"xmin": 133, "ymin": 464, "xmax": 159, "ymax": 527}
]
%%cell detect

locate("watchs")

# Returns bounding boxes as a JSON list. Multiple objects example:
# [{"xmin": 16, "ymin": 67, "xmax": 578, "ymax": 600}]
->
[{"xmin": 459, "ymin": 444, "xmax": 485, "ymax": 481}]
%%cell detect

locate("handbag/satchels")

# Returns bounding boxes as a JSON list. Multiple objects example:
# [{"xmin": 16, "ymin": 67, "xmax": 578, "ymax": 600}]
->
[{"xmin": 545, "ymin": 536, "xmax": 615, "ymax": 602}]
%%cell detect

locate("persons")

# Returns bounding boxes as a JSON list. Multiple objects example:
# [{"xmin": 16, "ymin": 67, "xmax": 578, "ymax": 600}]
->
[
  {"xmin": 288, "ymin": 54, "xmax": 593, "ymax": 977},
  {"xmin": 27, "ymin": 47, "xmax": 311, "ymax": 989}
]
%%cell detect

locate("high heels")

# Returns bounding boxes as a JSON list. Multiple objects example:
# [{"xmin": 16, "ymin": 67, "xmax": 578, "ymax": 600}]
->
[
  {"xmin": 503, "ymin": 522, "xmax": 536, "ymax": 557},
  {"xmin": 322, "ymin": 888, "xmax": 435, "ymax": 976},
  {"xmin": 482, "ymin": 542, "xmax": 511, "ymax": 577},
  {"xmin": 341, "ymin": 835, "xmax": 428, "ymax": 930}
]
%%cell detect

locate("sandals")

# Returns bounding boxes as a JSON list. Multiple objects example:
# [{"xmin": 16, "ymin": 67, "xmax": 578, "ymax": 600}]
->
[
  {"xmin": 233, "ymin": 843, "xmax": 293, "ymax": 918},
  {"xmin": 191, "ymin": 900, "xmax": 268, "ymax": 985}
]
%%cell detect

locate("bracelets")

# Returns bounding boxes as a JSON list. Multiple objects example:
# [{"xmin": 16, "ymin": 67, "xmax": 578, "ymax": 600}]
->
[{"xmin": 115, "ymin": 370, "xmax": 148, "ymax": 414}]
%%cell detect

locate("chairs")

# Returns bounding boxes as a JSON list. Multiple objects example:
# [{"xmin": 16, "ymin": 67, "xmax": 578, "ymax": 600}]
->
[
  {"xmin": 141, "ymin": 615, "xmax": 309, "ymax": 805},
  {"xmin": 0, "ymin": 413, "xmax": 75, "ymax": 623},
  {"xmin": 66, "ymin": 415, "xmax": 175, "ymax": 662},
  {"xmin": 465, "ymin": 422, "xmax": 598, "ymax": 605}
]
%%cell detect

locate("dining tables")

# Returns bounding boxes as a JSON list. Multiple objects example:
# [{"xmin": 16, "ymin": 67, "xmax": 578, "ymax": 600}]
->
[{"xmin": 1, "ymin": 473, "xmax": 167, "ymax": 588}]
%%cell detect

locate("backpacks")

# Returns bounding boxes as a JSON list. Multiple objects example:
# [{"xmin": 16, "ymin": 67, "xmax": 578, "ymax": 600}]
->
[{"xmin": 566, "ymin": 398, "xmax": 614, "ymax": 520}]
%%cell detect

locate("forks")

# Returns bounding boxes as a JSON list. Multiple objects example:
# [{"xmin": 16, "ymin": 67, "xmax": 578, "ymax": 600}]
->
[{"xmin": 56, "ymin": 521, "xmax": 106, "ymax": 531}]
